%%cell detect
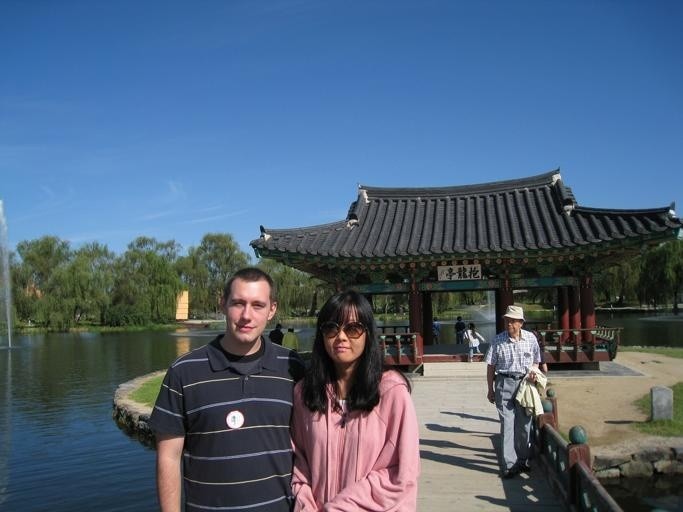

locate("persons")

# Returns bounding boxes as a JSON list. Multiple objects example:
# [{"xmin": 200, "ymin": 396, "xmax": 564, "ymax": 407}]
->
[
  {"xmin": 432, "ymin": 317, "xmax": 440, "ymax": 345},
  {"xmin": 149, "ymin": 268, "xmax": 306, "ymax": 512},
  {"xmin": 269, "ymin": 324, "xmax": 284, "ymax": 345},
  {"xmin": 531, "ymin": 329, "xmax": 547, "ymax": 373},
  {"xmin": 463, "ymin": 323, "xmax": 486, "ymax": 362},
  {"xmin": 483, "ymin": 305, "xmax": 542, "ymax": 479},
  {"xmin": 289, "ymin": 289, "xmax": 421, "ymax": 512},
  {"xmin": 455, "ymin": 317, "xmax": 465, "ymax": 344},
  {"xmin": 282, "ymin": 328, "xmax": 300, "ymax": 352}
]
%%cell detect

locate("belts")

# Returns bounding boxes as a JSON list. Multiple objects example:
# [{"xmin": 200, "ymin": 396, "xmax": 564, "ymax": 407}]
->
[{"xmin": 499, "ymin": 373, "xmax": 524, "ymax": 380}]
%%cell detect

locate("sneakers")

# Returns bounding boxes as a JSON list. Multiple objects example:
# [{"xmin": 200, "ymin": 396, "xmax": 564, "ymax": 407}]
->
[{"xmin": 503, "ymin": 464, "xmax": 531, "ymax": 479}]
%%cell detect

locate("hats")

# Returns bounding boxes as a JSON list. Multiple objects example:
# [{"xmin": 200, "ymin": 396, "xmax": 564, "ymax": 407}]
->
[{"xmin": 502, "ymin": 305, "xmax": 526, "ymax": 323}]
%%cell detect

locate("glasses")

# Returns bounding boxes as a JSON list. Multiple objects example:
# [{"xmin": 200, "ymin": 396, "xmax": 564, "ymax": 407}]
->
[{"xmin": 319, "ymin": 320, "xmax": 367, "ymax": 339}]
